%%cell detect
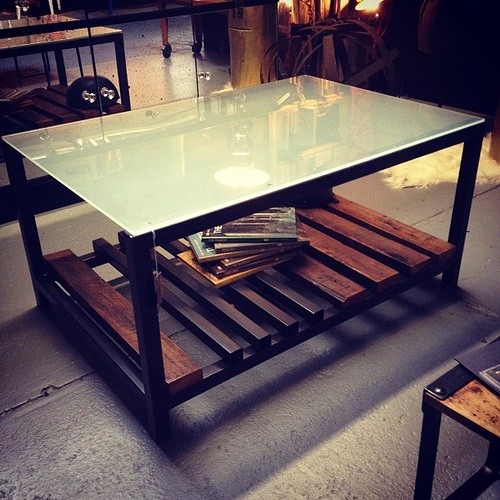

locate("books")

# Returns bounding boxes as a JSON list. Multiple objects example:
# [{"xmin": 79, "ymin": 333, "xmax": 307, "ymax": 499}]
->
[{"xmin": 184, "ymin": 206, "xmax": 310, "ymax": 288}]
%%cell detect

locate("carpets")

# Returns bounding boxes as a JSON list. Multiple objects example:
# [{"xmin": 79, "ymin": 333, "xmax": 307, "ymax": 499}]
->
[{"xmin": 380, "ymin": 134, "xmax": 500, "ymax": 190}]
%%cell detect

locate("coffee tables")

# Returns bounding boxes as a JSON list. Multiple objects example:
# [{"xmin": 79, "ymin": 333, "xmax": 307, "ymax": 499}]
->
[
  {"xmin": 0, "ymin": 25, "xmax": 132, "ymax": 191},
  {"xmin": 412, "ymin": 365, "xmax": 498, "ymax": 499},
  {"xmin": 1, "ymin": 75, "xmax": 493, "ymax": 443},
  {"xmin": 0, "ymin": 13, "xmax": 79, "ymax": 70}
]
